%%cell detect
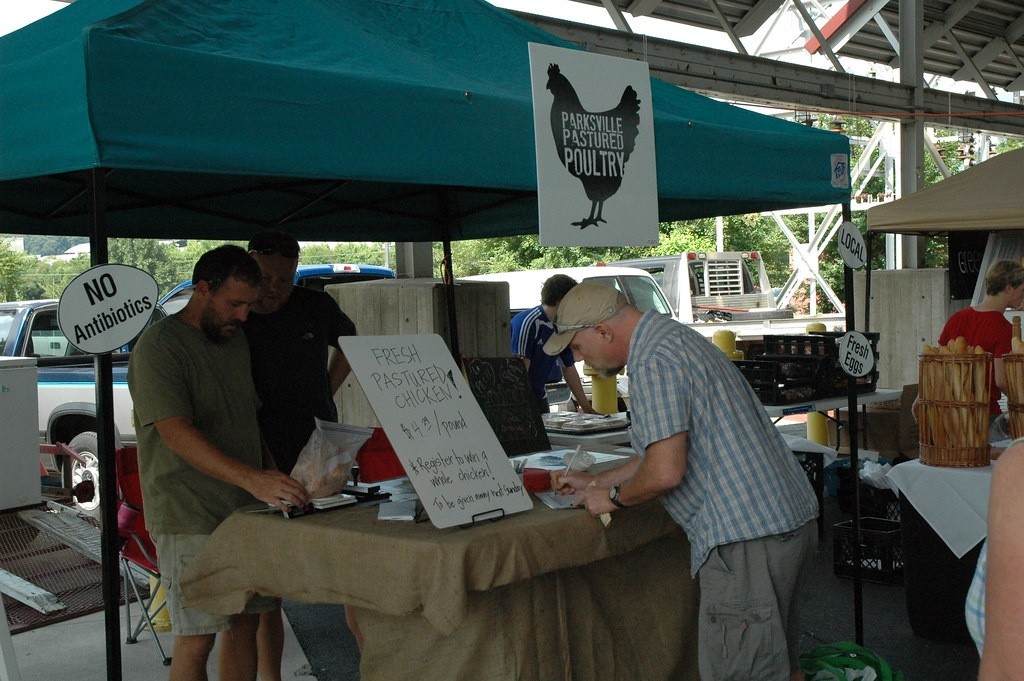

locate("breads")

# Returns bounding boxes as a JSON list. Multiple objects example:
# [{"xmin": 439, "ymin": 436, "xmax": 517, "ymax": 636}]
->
[
  {"xmin": 1006, "ymin": 336, "xmax": 1024, "ymax": 438},
  {"xmin": 918, "ymin": 335, "xmax": 989, "ymax": 449}
]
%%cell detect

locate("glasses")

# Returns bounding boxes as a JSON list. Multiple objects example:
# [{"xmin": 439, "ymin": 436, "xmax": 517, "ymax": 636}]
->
[{"xmin": 248, "ymin": 241, "xmax": 299, "ymax": 258}]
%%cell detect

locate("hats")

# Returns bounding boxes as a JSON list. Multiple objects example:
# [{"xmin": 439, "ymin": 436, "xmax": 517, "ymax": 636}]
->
[{"xmin": 541, "ymin": 282, "xmax": 629, "ymax": 356}]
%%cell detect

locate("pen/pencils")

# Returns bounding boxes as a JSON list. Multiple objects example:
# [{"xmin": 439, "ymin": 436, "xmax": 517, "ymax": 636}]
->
[
  {"xmin": 555, "ymin": 444, "xmax": 581, "ymax": 495},
  {"xmin": 520, "ymin": 458, "xmax": 528, "ymax": 473}
]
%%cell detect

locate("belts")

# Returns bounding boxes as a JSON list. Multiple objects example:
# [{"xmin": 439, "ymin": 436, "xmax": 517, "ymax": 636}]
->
[{"xmin": 552, "ymin": 316, "xmax": 595, "ymax": 334}]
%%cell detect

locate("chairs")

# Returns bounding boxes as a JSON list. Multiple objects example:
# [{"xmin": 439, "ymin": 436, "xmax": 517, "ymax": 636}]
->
[{"xmin": 111, "ymin": 445, "xmax": 174, "ymax": 665}]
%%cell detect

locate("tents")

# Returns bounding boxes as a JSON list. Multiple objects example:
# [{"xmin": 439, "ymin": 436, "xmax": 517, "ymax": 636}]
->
[
  {"xmin": 0, "ymin": 0, "xmax": 856, "ymax": 681},
  {"xmin": 864, "ymin": 146, "xmax": 1024, "ymax": 336}
]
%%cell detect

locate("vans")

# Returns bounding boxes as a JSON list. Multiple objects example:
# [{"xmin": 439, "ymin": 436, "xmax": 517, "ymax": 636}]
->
[
  {"xmin": 158, "ymin": 264, "xmax": 395, "ymax": 315},
  {"xmin": 456, "ymin": 267, "xmax": 679, "ymax": 418}
]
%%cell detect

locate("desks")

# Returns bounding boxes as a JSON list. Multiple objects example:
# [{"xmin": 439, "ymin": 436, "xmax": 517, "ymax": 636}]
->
[
  {"xmin": 177, "ymin": 439, "xmax": 702, "ymax": 681},
  {"xmin": 881, "ymin": 454, "xmax": 1002, "ymax": 641}
]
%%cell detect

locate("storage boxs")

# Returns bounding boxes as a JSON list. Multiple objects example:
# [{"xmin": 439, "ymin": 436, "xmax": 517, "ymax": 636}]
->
[
  {"xmin": 860, "ymin": 480, "xmax": 902, "ymax": 522},
  {"xmin": 792, "ymin": 451, "xmax": 825, "ymax": 508},
  {"xmin": 729, "ymin": 332, "xmax": 880, "ymax": 406},
  {"xmin": 832, "ymin": 516, "xmax": 904, "ymax": 585}
]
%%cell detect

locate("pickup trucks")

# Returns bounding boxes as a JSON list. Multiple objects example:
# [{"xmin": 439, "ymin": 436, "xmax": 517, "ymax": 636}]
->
[{"xmin": 0, "ymin": 299, "xmax": 172, "ymax": 521}]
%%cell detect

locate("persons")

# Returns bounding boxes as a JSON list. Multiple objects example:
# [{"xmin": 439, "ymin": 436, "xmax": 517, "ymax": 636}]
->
[
  {"xmin": 544, "ymin": 282, "xmax": 821, "ymax": 680},
  {"xmin": 123, "ymin": 246, "xmax": 309, "ymax": 681},
  {"xmin": 964, "ymin": 436, "xmax": 1024, "ymax": 681},
  {"xmin": 245, "ymin": 228, "xmax": 360, "ymax": 681},
  {"xmin": 911, "ymin": 261, "xmax": 1024, "ymax": 425},
  {"xmin": 510, "ymin": 274, "xmax": 600, "ymax": 415}
]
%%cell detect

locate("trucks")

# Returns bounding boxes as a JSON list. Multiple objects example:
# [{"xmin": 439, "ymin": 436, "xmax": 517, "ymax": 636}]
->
[{"xmin": 588, "ymin": 250, "xmax": 846, "ymax": 341}]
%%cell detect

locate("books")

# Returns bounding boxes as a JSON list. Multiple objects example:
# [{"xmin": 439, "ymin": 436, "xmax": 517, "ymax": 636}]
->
[
  {"xmin": 378, "ymin": 499, "xmax": 418, "ymax": 521},
  {"xmin": 309, "ymin": 494, "xmax": 358, "ymax": 509},
  {"xmin": 535, "ymin": 489, "xmax": 585, "ymax": 510}
]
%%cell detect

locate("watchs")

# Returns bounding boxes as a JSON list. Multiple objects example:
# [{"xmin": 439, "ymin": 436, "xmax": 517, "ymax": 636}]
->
[{"xmin": 608, "ymin": 485, "xmax": 627, "ymax": 510}]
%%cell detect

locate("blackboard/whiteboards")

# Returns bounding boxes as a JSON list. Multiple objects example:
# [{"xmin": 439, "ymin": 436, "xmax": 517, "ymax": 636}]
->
[{"xmin": 338, "ymin": 333, "xmax": 534, "ymax": 529}]
